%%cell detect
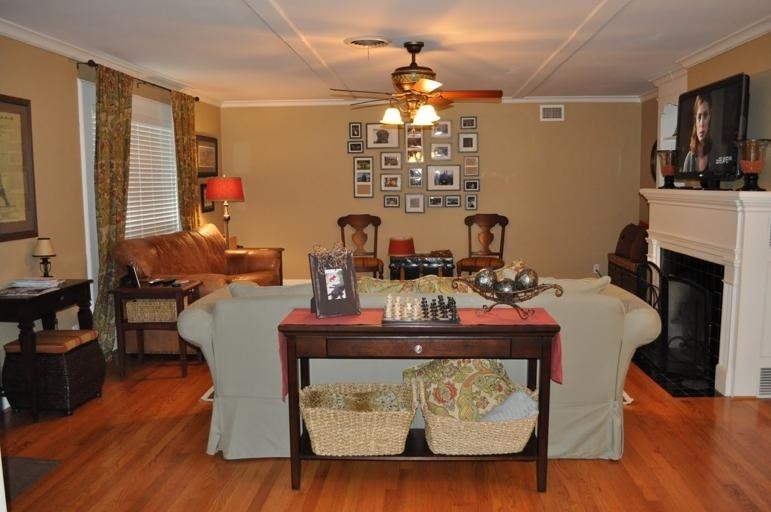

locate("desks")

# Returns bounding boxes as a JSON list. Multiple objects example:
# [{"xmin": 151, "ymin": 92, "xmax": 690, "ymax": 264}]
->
[
  {"xmin": 390, "ymin": 250, "xmax": 455, "ymax": 282},
  {"xmin": 0, "ymin": 276, "xmax": 96, "ymax": 423},
  {"xmin": 277, "ymin": 308, "xmax": 562, "ymax": 492}
]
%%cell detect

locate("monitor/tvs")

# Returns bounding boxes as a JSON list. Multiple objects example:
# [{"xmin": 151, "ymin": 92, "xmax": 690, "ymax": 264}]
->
[{"xmin": 673, "ymin": 72, "xmax": 750, "ymax": 190}]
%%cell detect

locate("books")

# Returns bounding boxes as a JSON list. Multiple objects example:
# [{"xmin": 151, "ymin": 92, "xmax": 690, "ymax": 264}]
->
[{"xmin": 0, "ymin": 272, "xmax": 61, "ymax": 299}]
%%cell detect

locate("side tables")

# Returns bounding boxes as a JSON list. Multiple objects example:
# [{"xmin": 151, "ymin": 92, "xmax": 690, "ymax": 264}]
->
[{"xmin": 110, "ymin": 276, "xmax": 203, "ymax": 379}]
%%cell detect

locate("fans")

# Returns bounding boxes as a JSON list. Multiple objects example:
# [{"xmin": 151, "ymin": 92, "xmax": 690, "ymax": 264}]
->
[{"xmin": 328, "ymin": 41, "xmax": 502, "ymax": 110}]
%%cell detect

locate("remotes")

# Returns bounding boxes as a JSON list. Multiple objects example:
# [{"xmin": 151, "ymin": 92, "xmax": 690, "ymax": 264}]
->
[
  {"xmin": 146, "ymin": 277, "xmax": 165, "ymax": 286},
  {"xmin": 162, "ymin": 277, "xmax": 176, "ymax": 286}
]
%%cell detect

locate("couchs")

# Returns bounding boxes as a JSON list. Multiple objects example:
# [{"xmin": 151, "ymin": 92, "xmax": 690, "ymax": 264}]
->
[
  {"xmin": 176, "ymin": 275, "xmax": 665, "ymax": 462},
  {"xmin": 107, "ymin": 223, "xmax": 286, "ymax": 356}
]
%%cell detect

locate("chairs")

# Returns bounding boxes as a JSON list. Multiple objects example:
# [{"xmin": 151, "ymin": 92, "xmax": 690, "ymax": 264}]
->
[
  {"xmin": 456, "ymin": 212, "xmax": 508, "ymax": 276},
  {"xmin": 335, "ymin": 214, "xmax": 384, "ymax": 280}
]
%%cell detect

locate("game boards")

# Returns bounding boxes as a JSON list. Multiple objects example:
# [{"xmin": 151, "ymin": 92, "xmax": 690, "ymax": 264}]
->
[{"xmin": 383, "ymin": 296, "xmax": 460, "ymax": 323}]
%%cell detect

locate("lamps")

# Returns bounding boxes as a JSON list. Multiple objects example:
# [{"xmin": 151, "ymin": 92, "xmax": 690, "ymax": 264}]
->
[
  {"xmin": 387, "ymin": 235, "xmax": 415, "ymax": 281},
  {"xmin": 30, "ymin": 236, "xmax": 59, "ymax": 279},
  {"xmin": 205, "ymin": 173, "xmax": 245, "ymax": 250},
  {"xmin": 380, "ymin": 94, "xmax": 441, "ymax": 132}
]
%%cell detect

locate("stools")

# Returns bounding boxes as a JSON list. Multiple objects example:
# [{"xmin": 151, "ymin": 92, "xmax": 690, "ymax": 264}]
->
[{"xmin": 3, "ymin": 330, "xmax": 106, "ymax": 418}]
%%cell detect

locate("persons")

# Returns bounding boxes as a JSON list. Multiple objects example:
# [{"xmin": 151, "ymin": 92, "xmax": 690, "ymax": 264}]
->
[{"xmin": 682, "ymin": 92, "xmax": 718, "ymax": 173}]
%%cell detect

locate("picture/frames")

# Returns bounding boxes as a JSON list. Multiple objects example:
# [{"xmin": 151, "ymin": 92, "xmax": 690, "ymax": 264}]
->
[
  {"xmin": 0, "ymin": 94, "xmax": 40, "ymax": 244},
  {"xmin": 346, "ymin": 115, "xmax": 481, "ymax": 214},
  {"xmin": 199, "ymin": 183, "xmax": 214, "ymax": 214},
  {"xmin": 196, "ymin": 135, "xmax": 219, "ymax": 177}
]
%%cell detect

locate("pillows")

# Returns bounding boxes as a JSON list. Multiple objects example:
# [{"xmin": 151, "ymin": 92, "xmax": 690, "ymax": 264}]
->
[
  {"xmin": 403, "ymin": 358, "xmax": 518, "ymax": 424},
  {"xmin": 227, "ymin": 280, "xmax": 316, "ymax": 297},
  {"xmin": 503, "ymin": 269, "xmax": 611, "ymax": 297}
]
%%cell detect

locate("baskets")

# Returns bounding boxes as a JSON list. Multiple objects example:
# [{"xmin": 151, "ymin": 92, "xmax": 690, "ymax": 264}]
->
[
  {"xmin": 298, "ymin": 376, "xmax": 417, "ymax": 457},
  {"xmin": 418, "ymin": 378, "xmax": 539, "ymax": 457},
  {"xmin": 126, "ymin": 296, "xmax": 189, "ymax": 322}
]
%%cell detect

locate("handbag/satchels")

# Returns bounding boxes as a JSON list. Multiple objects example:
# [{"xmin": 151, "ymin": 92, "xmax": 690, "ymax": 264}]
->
[{"xmin": 615, "ymin": 224, "xmax": 647, "ymax": 262}]
